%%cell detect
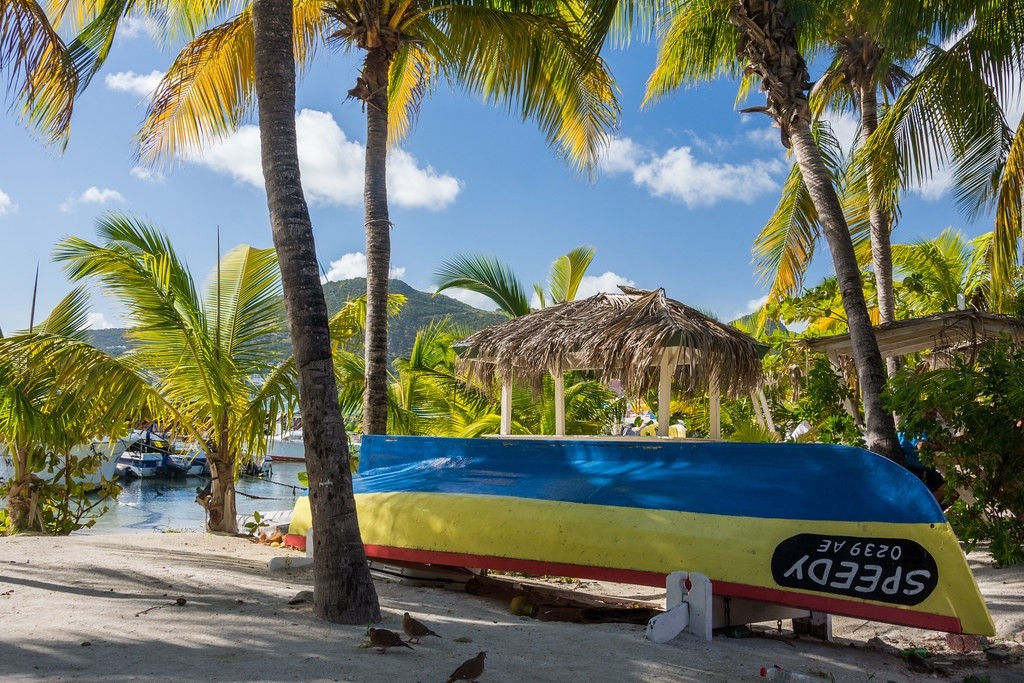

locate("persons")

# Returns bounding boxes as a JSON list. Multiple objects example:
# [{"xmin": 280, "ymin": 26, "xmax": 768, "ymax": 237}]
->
[{"xmin": 895, "ymin": 408, "xmax": 946, "ymax": 501}]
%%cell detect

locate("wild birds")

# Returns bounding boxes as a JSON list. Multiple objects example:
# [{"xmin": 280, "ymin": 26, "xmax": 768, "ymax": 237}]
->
[
  {"xmin": 446, "ymin": 652, "xmax": 487, "ymax": 683},
  {"xmin": 369, "ymin": 628, "xmax": 415, "ymax": 655},
  {"xmin": 402, "ymin": 612, "xmax": 442, "ymax": 645}
]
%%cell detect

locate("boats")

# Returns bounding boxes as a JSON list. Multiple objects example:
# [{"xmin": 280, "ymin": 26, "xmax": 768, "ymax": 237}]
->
[{"xmin": 0, "ymin": 406, "xmax": 362, "ymax": 497}]
